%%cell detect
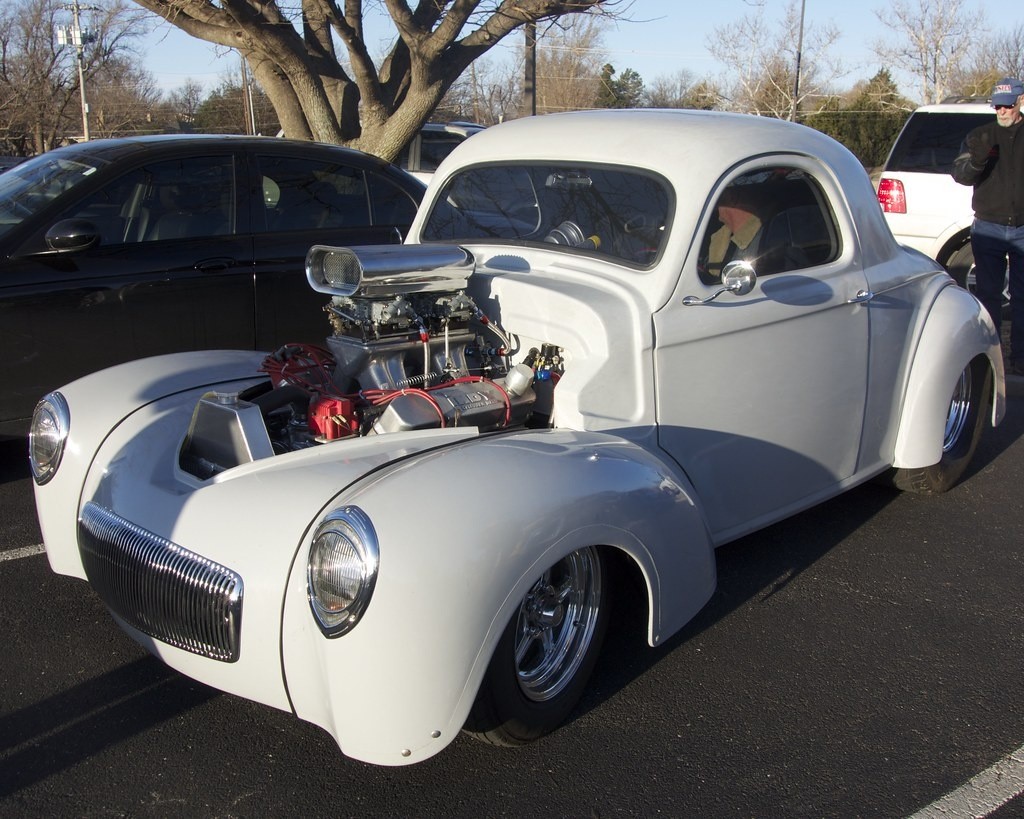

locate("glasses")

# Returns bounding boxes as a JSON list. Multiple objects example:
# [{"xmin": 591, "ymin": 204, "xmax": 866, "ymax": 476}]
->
[{"xmin": 995, "ymin": 105, "xmax": 1014, "ymax": 110}]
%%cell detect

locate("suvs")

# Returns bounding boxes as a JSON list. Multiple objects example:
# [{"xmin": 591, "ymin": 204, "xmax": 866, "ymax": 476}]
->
[{"xmin": 876, "ymin": 103, "xmax": 1024, "ymax": 314}]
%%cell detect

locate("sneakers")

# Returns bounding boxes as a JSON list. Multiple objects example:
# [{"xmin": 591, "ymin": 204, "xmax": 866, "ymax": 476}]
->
[{"xmin": 1011, "ymin": 355, "xmax": 1024, "ymax": 373}]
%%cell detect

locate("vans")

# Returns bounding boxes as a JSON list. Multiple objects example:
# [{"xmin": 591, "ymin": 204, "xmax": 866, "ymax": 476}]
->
[{"xmin": 277, "ymin": 123, "xmax": 490, "ymax": 205}]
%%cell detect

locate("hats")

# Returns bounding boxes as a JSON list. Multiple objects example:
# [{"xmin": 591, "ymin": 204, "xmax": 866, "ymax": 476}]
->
[{"xmin": 991, "ymin": 78, "xmax": 1023, "ymax": 105}]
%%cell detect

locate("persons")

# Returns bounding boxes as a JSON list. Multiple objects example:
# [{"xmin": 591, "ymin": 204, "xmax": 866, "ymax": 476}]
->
[
  {"xmin": 709, "ymin": 179, "xmax": 807, "ymax": 277},
  {"xmin": 952, "ymin": 77, "xmax": 1024, "ymax": 374}
]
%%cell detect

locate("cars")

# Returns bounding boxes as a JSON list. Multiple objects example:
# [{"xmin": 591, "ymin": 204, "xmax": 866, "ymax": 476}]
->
[
  {"xmin": 0, "ymin": 135, "xmax": 484, "ymax": 435},
  {"xmin": 27, "ymin": 112, "xmax": 1006, "ymax": 767}
]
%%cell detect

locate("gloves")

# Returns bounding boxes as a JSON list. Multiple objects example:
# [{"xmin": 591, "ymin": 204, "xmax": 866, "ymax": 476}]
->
[{"xmin": 967, "ymin": 139, "xmax": 996, "ymax": 165}]
